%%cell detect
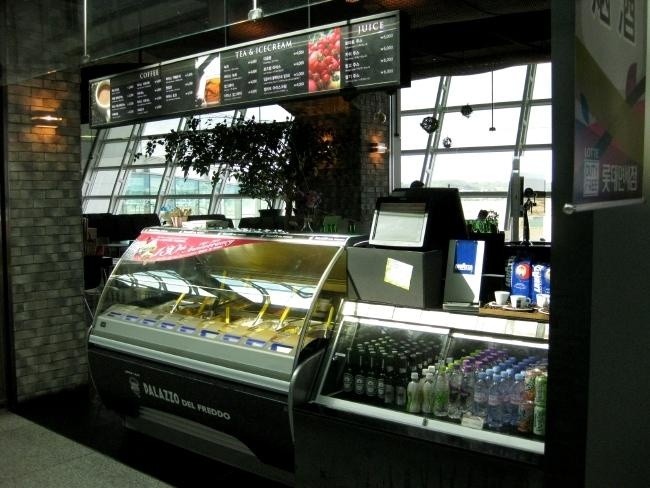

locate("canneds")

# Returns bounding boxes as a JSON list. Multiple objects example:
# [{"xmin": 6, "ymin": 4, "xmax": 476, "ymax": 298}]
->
[{"xmin": 516, "ymin": 367, "xmax": 549, "ymax": 436}]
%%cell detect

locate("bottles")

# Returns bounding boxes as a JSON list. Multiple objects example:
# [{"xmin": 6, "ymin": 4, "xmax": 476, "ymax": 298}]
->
[{"xmin": 345, "ymin": 336, "xmax": 547, "ymax": 430}]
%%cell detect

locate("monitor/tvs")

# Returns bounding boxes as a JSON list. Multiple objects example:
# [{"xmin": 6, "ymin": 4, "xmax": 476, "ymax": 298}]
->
[
  {"xmin": 393, "ymin": 186, "xmax": 469, "ymax": 243},
  {"xmin": 367, "ymin": 196, "xmax": 430, "ymax": 249}
]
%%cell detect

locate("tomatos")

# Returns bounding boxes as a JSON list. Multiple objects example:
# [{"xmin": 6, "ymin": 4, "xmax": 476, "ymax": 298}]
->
[{"xmin": 307, "ymin": 28, "xmax": 341, "ymax": 92}]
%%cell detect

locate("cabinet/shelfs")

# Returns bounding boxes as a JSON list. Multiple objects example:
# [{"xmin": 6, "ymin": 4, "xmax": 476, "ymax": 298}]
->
[
  {"xmin": 84, "ymin": 225, "xmax": 372, "ymax": 488},
  {"xmin": 301, "ymin": 295, "xmax": 551, "ymax": 465}
]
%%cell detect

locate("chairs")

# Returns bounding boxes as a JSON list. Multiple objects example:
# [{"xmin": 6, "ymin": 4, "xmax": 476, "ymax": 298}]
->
[{"xmin": 84, "ymin": 255, "xmax": 115, "ymax": 321}]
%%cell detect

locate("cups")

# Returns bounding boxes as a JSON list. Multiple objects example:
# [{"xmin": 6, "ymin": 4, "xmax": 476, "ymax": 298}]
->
[
  {"xmin": 489, "ymin": 289, "xmax": 549, "ymax": 315},
  {"xmin": 159, "ymin": 211, "xmax": 172, "ymax": 225},
  {"xmin": 95, "ymin": 80, "xmax": 111, "ymax": 122},
  {"xmin": 203, "ymin": 78, "xmax": 220, "ymax": 104}
]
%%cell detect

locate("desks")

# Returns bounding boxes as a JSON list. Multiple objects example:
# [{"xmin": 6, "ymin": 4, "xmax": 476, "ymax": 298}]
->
[{"xmin": 100, "ymin": 241, "xmax": 131, "ymax": 267}]
